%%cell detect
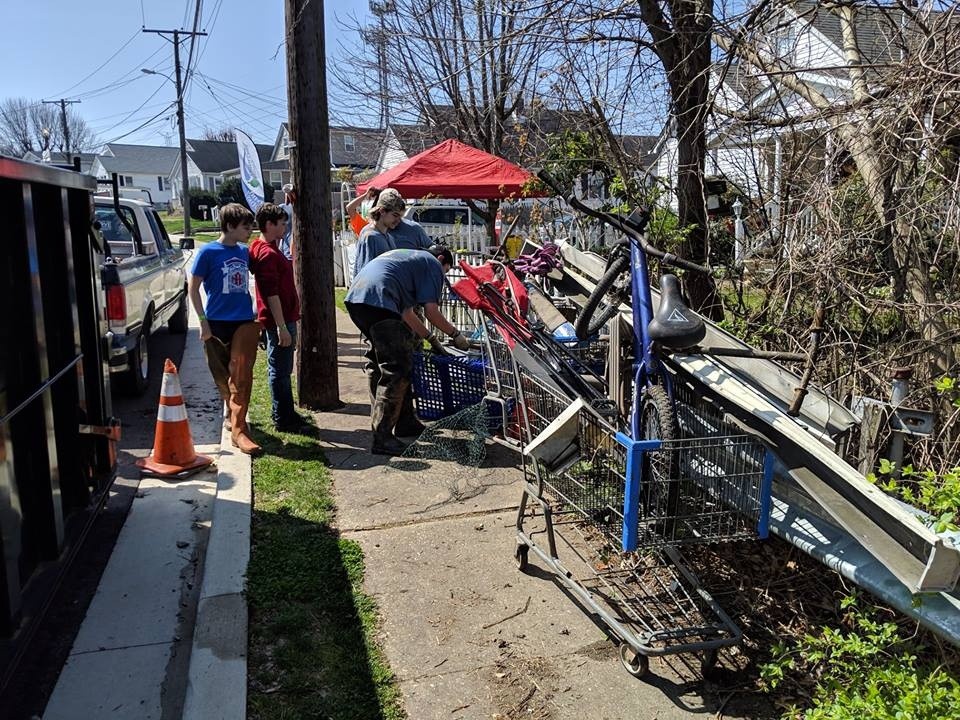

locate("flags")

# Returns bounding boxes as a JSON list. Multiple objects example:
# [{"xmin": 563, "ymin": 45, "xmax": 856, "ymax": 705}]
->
[{"xmin": 234, "ymin": 128, "xmax": 265, "ymax": 214}]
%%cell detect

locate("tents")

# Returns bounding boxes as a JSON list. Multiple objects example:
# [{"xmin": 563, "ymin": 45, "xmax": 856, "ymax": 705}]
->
[{"xmin": 357, "ymin": 139, "xmax": 550, "ymax": 199}]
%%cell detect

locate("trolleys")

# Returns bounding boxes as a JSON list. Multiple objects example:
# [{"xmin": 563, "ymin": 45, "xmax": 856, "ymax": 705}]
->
[{"xmin": 461, "ymin": 293, "xmax": 770, "ymax": 678}]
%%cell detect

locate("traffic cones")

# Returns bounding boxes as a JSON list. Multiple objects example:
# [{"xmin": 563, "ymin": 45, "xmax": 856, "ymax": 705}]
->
[{"xmin": 128, "ymin": 359, "xmax": 212, "ymax": 479}]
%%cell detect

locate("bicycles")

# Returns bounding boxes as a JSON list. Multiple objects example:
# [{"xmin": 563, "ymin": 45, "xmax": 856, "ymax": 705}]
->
[{"xmin": 533, "ymin": 167, "xmax": 709, "ymax": 545}]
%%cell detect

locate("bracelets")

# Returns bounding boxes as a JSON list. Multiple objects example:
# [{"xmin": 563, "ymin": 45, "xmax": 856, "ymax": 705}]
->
[
  {"xmin": 197, "ymin": 313, "xmax": 205, "ymax": 320},
  {"xmin": 277, "ymin": 322, "xmax": 288, "ymax": 331}
]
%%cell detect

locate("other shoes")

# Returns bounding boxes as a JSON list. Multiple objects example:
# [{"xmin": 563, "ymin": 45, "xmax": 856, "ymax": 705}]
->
[
  {"xmin": 271, "ymin": 410, "xmax": 312, "ymax": 424},
  {"xmin": 275, "ymin": 419, "xmax": 320, "ymax": 434}
]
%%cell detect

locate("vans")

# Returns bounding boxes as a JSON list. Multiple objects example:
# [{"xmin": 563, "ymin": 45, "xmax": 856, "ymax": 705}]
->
[{"xmin": 400, "ymin": 206, "xmax": 502, "ymax": 252}]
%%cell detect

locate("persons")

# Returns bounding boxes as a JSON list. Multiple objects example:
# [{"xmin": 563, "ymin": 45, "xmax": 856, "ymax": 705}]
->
[
  {"xmin": 387, "ymin": 208, "xmax": 436, "ymax": 388},
  {"xmin": 353, "ymin": 188, "xmax": 405, "ymax": 277},
  {"xmin": 346, "ymin": 186, "xmax": 382, "ymax": 236},
  {"xmin": 343, "ymin": 244, "xmax": 471, "ymax": 457},
  {"xmin": 249, "ymin": 202, "xmax": 313, "ymax": 432},
  {"xmin": 277, "ymin": 184, "xmax": 292, "ymax": 261},
  {"xmin": 188, "ymin": 204, "xmax": 261, "ymax": 453}
]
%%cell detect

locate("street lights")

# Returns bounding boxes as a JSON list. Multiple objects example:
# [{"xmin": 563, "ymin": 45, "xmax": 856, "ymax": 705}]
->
[{"xmin": 141, "ymin": 67, "xmax": 196, "ymax": 253}]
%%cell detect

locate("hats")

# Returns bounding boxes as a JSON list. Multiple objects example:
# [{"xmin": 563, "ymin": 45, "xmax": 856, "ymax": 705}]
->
[
  {"xmin": 369, "ymin": 192, "xmax": 405, "ymax": 213},
  {"xmin": 419, "ymin": 243, "xmax": 455, "ymax": 267}
]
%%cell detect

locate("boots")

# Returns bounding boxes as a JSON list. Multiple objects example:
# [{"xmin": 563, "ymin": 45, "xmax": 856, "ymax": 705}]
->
[
  {"xmin": 228, "ymin": 323, "xmax": 259, "ymax": 454},
  {"xmin": 371, "ymin": 319, "xmax": 422, "ymax": 459},
  {"xmin": 363, "ymin": 335, "xmax": 432, "ymax": 438},
  {"xmin": 202, "ymin": 335, "xmax": 233, "ymax": 431}
]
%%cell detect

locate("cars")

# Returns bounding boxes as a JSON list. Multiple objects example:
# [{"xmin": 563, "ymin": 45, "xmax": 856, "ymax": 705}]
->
[{"xmin": 537, "ymin": 215, "xmax": 599, "ymax": 242}]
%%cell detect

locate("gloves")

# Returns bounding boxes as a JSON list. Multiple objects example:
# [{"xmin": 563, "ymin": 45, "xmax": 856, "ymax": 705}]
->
[
  {"xmin": 450, "ymin": 330, "xmax": 470, "ymax": 350},
  {"xmin": 424, "ymin": 335, "xmax": 449, "ymax": 356}
]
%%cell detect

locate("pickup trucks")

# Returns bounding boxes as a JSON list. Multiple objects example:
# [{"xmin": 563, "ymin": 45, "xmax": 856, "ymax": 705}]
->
[{"xmin": 92, "ymin": 186, "xmax": 194, "ymax": 390}]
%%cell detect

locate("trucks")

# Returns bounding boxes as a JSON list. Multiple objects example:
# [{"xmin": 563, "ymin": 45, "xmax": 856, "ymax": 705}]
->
[{"xmin": 0, "ymin": 152, "xmax": 124, "ymax": 656}]
